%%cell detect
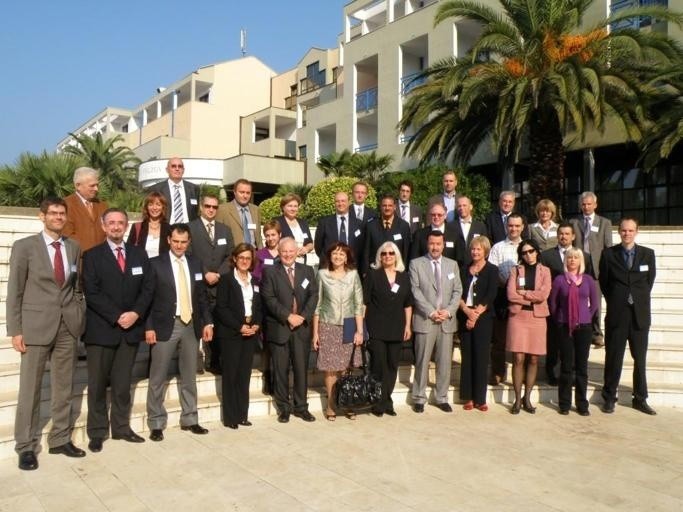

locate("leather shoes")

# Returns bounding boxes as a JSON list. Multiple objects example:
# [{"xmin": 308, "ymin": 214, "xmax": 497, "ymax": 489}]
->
[
  {"xmin": 294, "ymin": 410, "xmax": 315, "ymax": 421},
  {"xmin": 438, "ymin": 403, "xmax": 452, "ymax": 412},
  {"xmin": 632, "ymin": 399, "xmax": 656, "ymax": 415},
  {"xmin": 18, "ymin": 451, "xmax": 38, "ymax": 470},
  {"xmin": 225, "ymin": 424, "xmax": 238, "ymax": 429},
  {"xmin": 592, "ymin": 336, "xmax": 604, "ymax": 347},
  {"xmin": 549, "ymin": 378, "xmax": 557, "ymax": 386},
  {"xmin": 415, "ymin": 403, "xmax": 424, "ymax": 412},
  {"xmin": 112, "ymin": 430, "xmax": 145, "ymax": 443},
  {"xmin": 384, "ymin": 409, "xmax": 396, "ymax": 416},
  {"xmin": 49, "ymin": 442, "xmax": 86, "ymax": 458},
  {"xmin": 602, "ymin": 401, "xmax": 615, "ymax": 413},
  {"xmin": 181, "ymin": 424, "xmax": 209, "ymax": 434},
  {"xmin": 240, "ymin": 421, "xmax": 252, "ymax": 426},
  {"xmin": 206, "ymin": 366, "xmax": 223, "ymax": 375},
  {"xmin": 560, "ymin": 406, "xmax": 569, "ymax": 414},
  {"xmin": 150, "ymin": 429, "xmax": 164, "ymax": 441},
  {"xmin": 88, "ymin": 438, "xmax": 102, "ymax": 452},
  {"xmin": 278, "ymin": 411, "xmax": 290, "ymax": 423},
  {"xmin": 577, "ymin": 407, "xmax": 589, "ymax": 416},
  {"xmin": 372, "ymin": 410, "xmax": 384, "ymax": 416},
  {"xmin": 197, "ymin": 368, "xmax": 204, "ymax": 374}
]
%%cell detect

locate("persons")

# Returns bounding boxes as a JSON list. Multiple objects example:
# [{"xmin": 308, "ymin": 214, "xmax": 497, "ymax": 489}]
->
[
  {"xmin": 441, "ymin": 169, "xmax": 462, "ymax": 225},
  {"xmin": 81, "ymin": 208, "xmax": 157, "ymax": 453},
  {"xmin": 483, "ymin": 190, "xmax": 529, "ymax": 244},
  {"xmin": 527, "ymin": 199, "xmax": 560, "ymax": 253},
  {"xmin": 538, "ymin": 223, "xmax": 591, "ymax": 385},
  {"xmin": 546, "ymin": 248, "xmax": 601, "ymax": 416},
  {"xmin": 363, "ymin": 195, "xmax": 410, "ymax": 303},
  {"xmin": 183, "ymin": 196, "xmax": 234, "ymax": 377},
  {"xmin": 568, "ymin": 190, "xmax": 612, "ymax": 346},
  {"xmin": 271, "ymin": 191, "xmax": 314, "ymax": 267},
  {"xmin": 504, "ymin": 239, "xmax": 551, "ymax": 414},
  {"xmin": 446, "ymin": 195, "xmax": 488, "ymax": 269},
  {"xmin": 316, "ymin": 191, "xmax": 366, "ymax": 281},
  {"xmin": 214, "ymin": 178, "xmax": 262, "ymax": 251},
  {"xmin": 143, "ymin": 223, "xmax": 214, "ymax": 442},
  {"xmin": 251, "ymin": 220, "xmax": 282, "ymax": 281},
  {"xmin": 364, "ymin": 241, "xmax": 414, "ymax": 417},
  {"xmin": 487, "ymin": 213, "xmax": 525, "ymax": 386},
  {"xmin": 311, "ymin": 242, "xmax": 364, "ymax": 420},
  {"xmin": 459, "ymin": 236, "xmax": 498, "ymax": 411},
  {"xmin": 5, "ymin": 197, "xmax": 87, "ymax": 471},
  {"xmin": 125, "ymin": 192, "xmax": 173, "ymax": 377},
  {"xmin": 216, "ymin": 242, "xmax": 263, "ymax": 429},
  {"xmin": 597, "ymin": 216, "xmax": 656, "ymax": 416},
  {"xmin": 58, "ymin": 166, "xmax": 109, "ymax": 360},
  {"xmin": 263, "ymin": 236, "xmax": 319, "ymax": 424},
  {"xmin": 410, "ymin": 194, "xmax": 466, "ymax": 363},
  {"xmin": 150, "ymin": 157, "xmax": 200, "ymax": 225},
  {"xmin": 408, "ymin": 230, "xmax": 462, "ymax": 413},
  {"xmin": 347, "ymin": 181, "xmax": 378, "ymax": 226},
  {"xmin": 393, "ymin": 180, "xmax": 422, "ymax": 238}
]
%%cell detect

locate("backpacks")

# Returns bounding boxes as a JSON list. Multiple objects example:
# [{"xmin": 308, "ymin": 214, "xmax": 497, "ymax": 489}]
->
[{"xmin": 492, "ymin": 265, "xmax": 520, "ymax": 323}]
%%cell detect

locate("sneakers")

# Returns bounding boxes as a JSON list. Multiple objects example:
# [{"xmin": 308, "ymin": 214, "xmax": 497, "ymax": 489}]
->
[{"xmin": 489, "ymin": 374, "xmax": 500, "ymax": 385}]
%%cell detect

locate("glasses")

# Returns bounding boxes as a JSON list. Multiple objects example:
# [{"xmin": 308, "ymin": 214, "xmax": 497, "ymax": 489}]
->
[
  {"xmin": 202, "ymin": 204, "xmax": 219, "ymax": 210},
  {"xmin": 381, "ymin": 252, "xmax": 395, "ymax": 256},
  {"xmin": 430, "ymin": 214, "xmax": 445, "ymax": 217},
  {"xmin": 235, "ymin": 256, "xmax": 252, "ymax": 260},
  {"xmin": 169, "ymin": 165, "xmax": 183, "ymax": 168},
  {"xmin": 521, "ymin": 249, "xmax": 536, "ymax": 255}
]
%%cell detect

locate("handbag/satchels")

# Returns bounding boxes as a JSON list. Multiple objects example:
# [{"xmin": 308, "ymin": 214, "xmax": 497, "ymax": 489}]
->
[{"xmin": 335, "ymin": 343, "xmax": 383, "ymax": 409}]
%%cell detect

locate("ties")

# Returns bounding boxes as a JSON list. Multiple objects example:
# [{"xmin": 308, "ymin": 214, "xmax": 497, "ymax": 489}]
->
[
  {"xmin": 86, "ymin": 201, "xmax": 93, "ymax": 220},
  {"xmin": 584, "ymin": 217, "xmax": 591, "ymax": 252},
  {"xmin": 626, "ymin": 251, "xmax": 633, "ymax": 271},
  {"xmin": 433, "ymin": 261, "xmax": 442, "ymax": 311},
  {"xmin": 357, "ymin": 207, "xmax": 362, "ymax": 220},
  {"xmin": 504, "ymin": 216, "xmax": 508, "ymax": 237},
  {"xmin": 401, "ymin": 205, "xmax": 408, "ymax": 222},
  {"xmin": 207, "ymin": 222, "xmax": 214, "ymax": 241},
  {"xmin": 50, "ymin": 242, "xmax": 65, "ymax": 288},
  {"xmin": 385, "ymin": 222, "xmax": 390, "ymax": 230},
  {"xmin": 287, "ymin": 268, "xmax": 297, "ymax": 314},
  {"xmin": 340, "ymin": 217, "xmax": 347, "ymax": 245},
  {"xmin": 174, "ymin": 185, "xmax": 184, "ymax": 223},
  {"xmin": 241, "ymin": 208, "xmax": 251, "ymax": 245},
  {"xmin": 563, "ymin": 251, "xmax": 568, "ymax": 261},
  {"xmin": 116, "ymin": 247, "xmax": 125, "ymax": 273},
  {"xmin": 176, "ymin": 259, "xmax": 192, "ymax": 324}
]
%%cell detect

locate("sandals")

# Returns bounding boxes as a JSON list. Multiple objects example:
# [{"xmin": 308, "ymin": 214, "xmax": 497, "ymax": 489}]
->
[
  {"xmin": 463, "ymin": 403, "xmax": 473, "ymax": 410},
  {"xmin": 344, "ymin": 409, "xmax": 356, "ymax": 419},
  {"xmin": 479, "ymin": 404, "xmax": 488, "ymax": 411},
  {"xmin": 327, "ymin": 408, "xmax": 336, "ymax": 421}
]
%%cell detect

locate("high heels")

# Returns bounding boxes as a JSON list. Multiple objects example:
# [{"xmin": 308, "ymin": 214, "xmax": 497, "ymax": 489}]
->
[
  {"xmin": 522, "ymin": 397, "xmax": 535, "ymax": 414},
  {"xmin": 511, "ymin": 399, "xmax": 523, "ymax": 414}
]
